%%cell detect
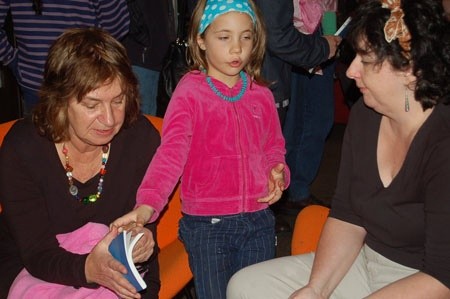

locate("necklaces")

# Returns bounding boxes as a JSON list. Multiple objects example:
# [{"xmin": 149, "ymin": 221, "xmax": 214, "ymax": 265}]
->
[
  {"xmin": 58, "ymin": 143, "xmax": 109, "ymax": 203},
  {"xmin": 201, "ymin": 68, "xmax": 251, "ymax": 101}
]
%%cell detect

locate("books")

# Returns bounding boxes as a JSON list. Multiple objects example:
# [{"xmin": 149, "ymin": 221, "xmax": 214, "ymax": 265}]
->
[{"xmin": 107, "ymin": 230, "xmax": 147, "ymax": 295}]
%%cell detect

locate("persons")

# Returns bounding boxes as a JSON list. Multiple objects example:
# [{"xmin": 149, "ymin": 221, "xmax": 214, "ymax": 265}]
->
[
  {"xmin": 0, "ymin": 0, "xmax": 450, "ymax": 299},
  {"xmin": 0, "ymin": 29, "xmax": 161, "ymax": 299},
  {"xmin": 226, "ymin": 1, "xmax": 450, "ymax": 299}
]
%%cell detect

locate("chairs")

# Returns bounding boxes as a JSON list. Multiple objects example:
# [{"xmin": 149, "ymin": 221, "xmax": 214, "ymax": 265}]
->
[{"xmin": 0, "ymin": 114, "xmax": 196, "ymax": 299}]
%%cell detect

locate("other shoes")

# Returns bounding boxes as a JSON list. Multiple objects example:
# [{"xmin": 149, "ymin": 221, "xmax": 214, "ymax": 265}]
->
[
  {"xmin": 274, "ymin": 214, "xmax": 291, "ymax": 232},
  {"xmin": 284, "ymin": 194, "xmax": 332, "ymax": 217}
]
